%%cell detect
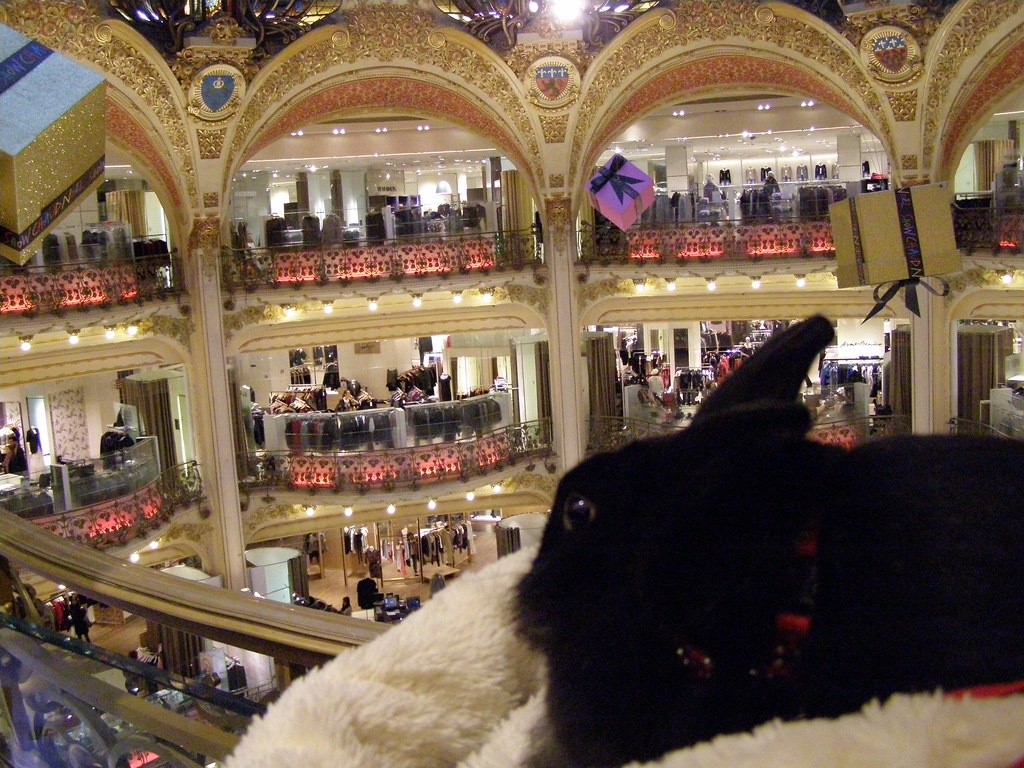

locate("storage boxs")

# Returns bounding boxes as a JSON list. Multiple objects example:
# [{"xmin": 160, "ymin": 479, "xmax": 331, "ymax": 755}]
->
[
  {"xmin": 829, "ymin": 182, "xmax": 964, "ymax": 324},
  {"xmin": 585, "ymin": 153, "xmax": 657, "ymax": 232}
]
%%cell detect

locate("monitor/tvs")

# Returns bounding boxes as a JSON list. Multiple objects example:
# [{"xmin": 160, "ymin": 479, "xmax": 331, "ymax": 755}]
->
[{"xmin": 384, "ymin": 596, "xmax": 399, "ymax": 611}]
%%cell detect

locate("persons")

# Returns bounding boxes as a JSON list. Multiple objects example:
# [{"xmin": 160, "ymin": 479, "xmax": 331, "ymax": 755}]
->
[
  {"xmin": 649, "ymin": 369, "xmax": 664, "ymax": 395},
  {"xmin": 742, "ymin": 337, "xmax": 753, "ymax": 355},
  {"xmin": 720, "ymin": 167, "xmax": 731, "ymax": 186},
  {"xmin": 704, "ymin": 174, "xmax": 722, "ymax": 202},
  {"xmin": 746, "ymin": 165, "xmax": 771, "ymax": 184},
  {"xmin": 194, "ymin": 677, "xmax": 220, "ymax": 706},
  {"xmin": 814, "ymin": 162, "xmax": 827, "ymax": 180},
  {"xmin": 832, "ymin": 162, "xmax": 839, "ymax": 178},
  {"xmin": 365, "ymin": 546, "xmax": 381, "ymax": 578},
  {"xmin": 796, "ymin": 164, "xmax": 809, "ymax": 181},
  {"xmin": 764, "ymin": 171, "xmax": 780, "ymax": 198},
  {"xmin": 337, "ymin": 390, "xmax": 352, "ymax": 411},
  {"xmin": 781, "ymin": 164, "xmax": 792, "ymax": 182},
  {"xmin": 26, "ymin": 426, "xmax": 44, "ymax": 480},
  {"xmin": 439, "ymin": 373, "xmax": 451, "ymax": 401},
  {"xmin": 12, "ymin": 428, "xmax": 20, "ymax": 441},
  {"xmin": 123, "ymin": 650, "xmax": 139, "ymax": 694},
  {"xmin": 339, "ymin": 597, "xmax": 352, "ymax": 615},
  {"xmin": 348, "ymin": 380, "xmax": 360, "ymax": 396},
  {"xmin": 630, "ymin": 338, "xmax": 640, "ymax": 349},
  {"xmin": 71, "ymin": 596, "xmax": 91, "ymax": 643},
  {"xmin": 6, "ymin": 436, "xmax": 27, "ymax": 472}
]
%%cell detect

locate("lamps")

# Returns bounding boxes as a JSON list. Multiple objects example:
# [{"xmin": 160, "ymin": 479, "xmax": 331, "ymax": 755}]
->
[
  {"xmin": 995, "ymin": 269, "xmax": 1014, "ymax": 284},
  {"xmin": 103, "ymin": 325, "xmax": 117, "ymax": 339},
  {"xmin": 410, "ymin": 293, "xmax": 423, "ymax": 306},
  {"xmin": 322, "ymin": 300, "xmax": 333, "ymax": 312},
  {"xmin": 750, "ymin": 276, "xmax": 762, "ymax": 289},
  {"xmin": 123, "ymin": 320, "xmax": 138, "ymax": 336},
  {"xmin": 664, "ymin": 278, "xmax": 676, "ymax": 290},
  {"xmin": 67, "ymin": 329, "xmax": 80, "ymax": 343},
  {"xmin": 18, "ymin": 335, "xmax": 34, "ymax": 352},
  {"xmin": 705, "ymin": 278, "xmax": 718, "ymax": 290},
  {"xmin": 451, "ymin": 291, "xmax": 463, "ymax": 304},
  {"xmin": 57, "ymin": 480, "xmax": 504, "ymax": 589},
  {"xmin": 633, "ymin": 279, "xmax": 647, "ymax": 292},
  {"xmin": 794, "ymin": 274, "xmax": 806, "ymax": 287},
  {"xmin": 366, "ymin": 297, "xmax": 379, "ymax": 310},
  {"xmin": 479, "ymin": 288, "xmax": 494, "ymax": 303}
]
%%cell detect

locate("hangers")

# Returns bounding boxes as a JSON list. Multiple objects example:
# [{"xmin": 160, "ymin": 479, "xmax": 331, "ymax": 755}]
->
[
  {"xmin": 659, "ymin": 331, "xmax": 884, "ymax": 373},
  {"xmin": 269, "ymin": 363, "xmax": 489, "ymax": 423}
]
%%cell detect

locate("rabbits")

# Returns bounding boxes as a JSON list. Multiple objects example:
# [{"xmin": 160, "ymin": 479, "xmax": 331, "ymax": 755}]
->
[{"xmin": 501, "ymin": 314, "xmax": 1024, "ymax": 767}]
